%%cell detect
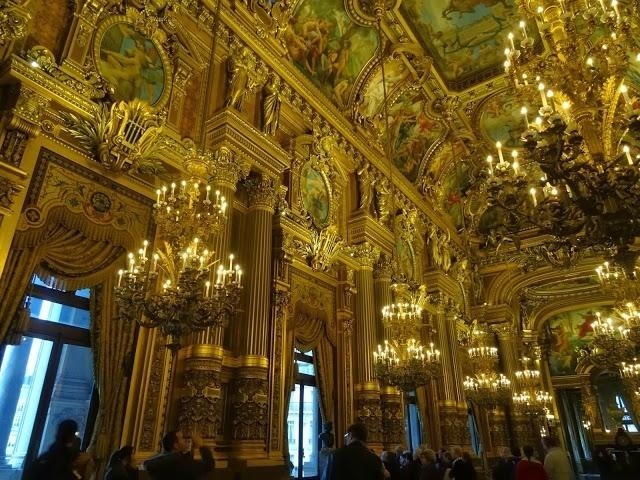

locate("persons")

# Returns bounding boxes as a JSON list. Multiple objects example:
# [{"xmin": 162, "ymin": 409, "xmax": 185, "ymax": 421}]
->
[
  {"xmin": 378, "ymin": 434, "xmax": 573, "ymax": 479},
  {"xmin": 317, "ymin": 421, "xmax": 334, "ymax": 449},
  {"xmin": 143, "ymin": 426, "xmax": 216, "ymax": 479},
  {"xmin": 104, "ymin": 444, "xmax": 140, "ymax": 479},
  {"xmin": 97, "ymin": 36, "xmax": 146, "ymax": 100},
  {"xmin": 72, "ymin": 451, "xmax": 95, "ymax": 480},
  {"xmin": 18, "ymin": 418, "xmax": 82, "ymax": 480},
  {"xmin": 594, "ymin": 427, "xmax": 640, "ymax": 480},
  {"xmin": 321, "ymin": 423, "xmax": 384, "ymax": 480}
]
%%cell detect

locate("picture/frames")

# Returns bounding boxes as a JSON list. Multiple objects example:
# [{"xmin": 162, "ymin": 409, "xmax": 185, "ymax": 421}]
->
[
  {"xmin": 299, "ymin": 160, "xmax": 333, "ymax": 229},
  {"xmin": 396, "ymin": 235, "xmax": 416, "ymax": 283},
  {"xmin": 92, "ymin": 14, "xmax": 173, "ymax": 114}
]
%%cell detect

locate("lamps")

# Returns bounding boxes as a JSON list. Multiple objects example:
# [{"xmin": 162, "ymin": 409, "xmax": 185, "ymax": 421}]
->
[
  {"xmin": 360, "ymin": 0, "xmax": 443, "ymax": 391},
  {"xmin": 113, "ymin": 1, "xmax": 242, "ymax": 355},
  {"xmin": 432, "ymin": 95, "xmax": 511, "ymax": 410}
]
